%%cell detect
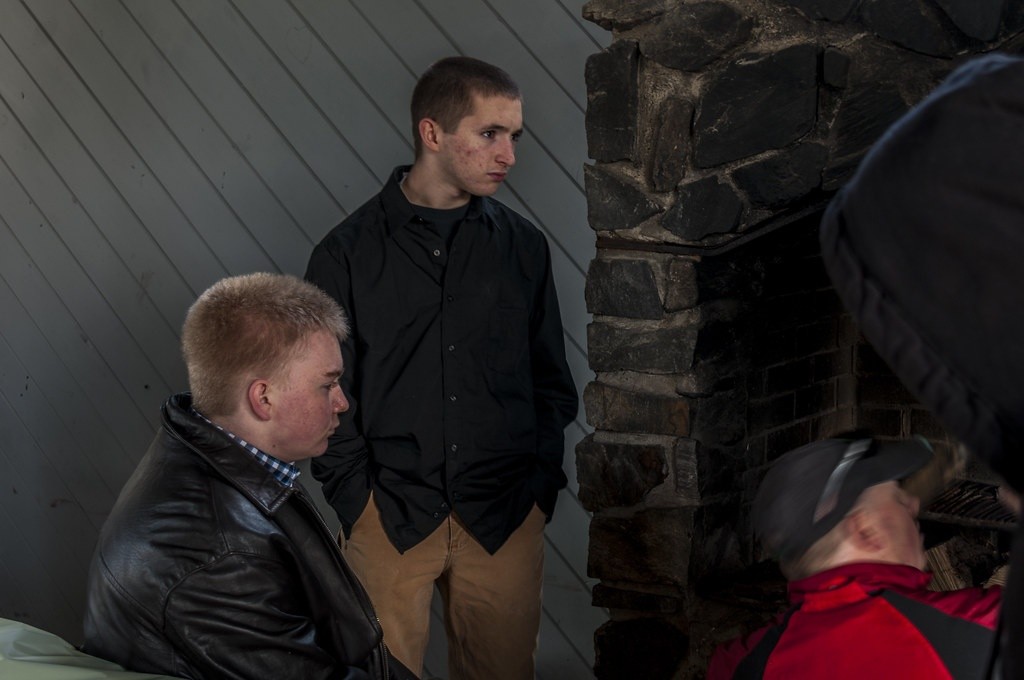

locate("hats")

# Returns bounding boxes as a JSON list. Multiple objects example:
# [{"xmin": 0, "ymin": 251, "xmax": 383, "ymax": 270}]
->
[{"xmin": 752, "ymin": 432, "xmax": 926, "ymax": 563}]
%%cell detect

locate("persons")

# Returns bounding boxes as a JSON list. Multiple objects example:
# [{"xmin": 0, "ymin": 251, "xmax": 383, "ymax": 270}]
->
[
  {"xmin": 306, "ymin": 55, "xmax": 582, "ymax": 680},
  {"xmin": 703, "ymin": 431, "xmax": 1024, "ymax": 680},
  {"xmin": 78, "ymin": 269, "xmax": 430, "ymax": 680}
]
